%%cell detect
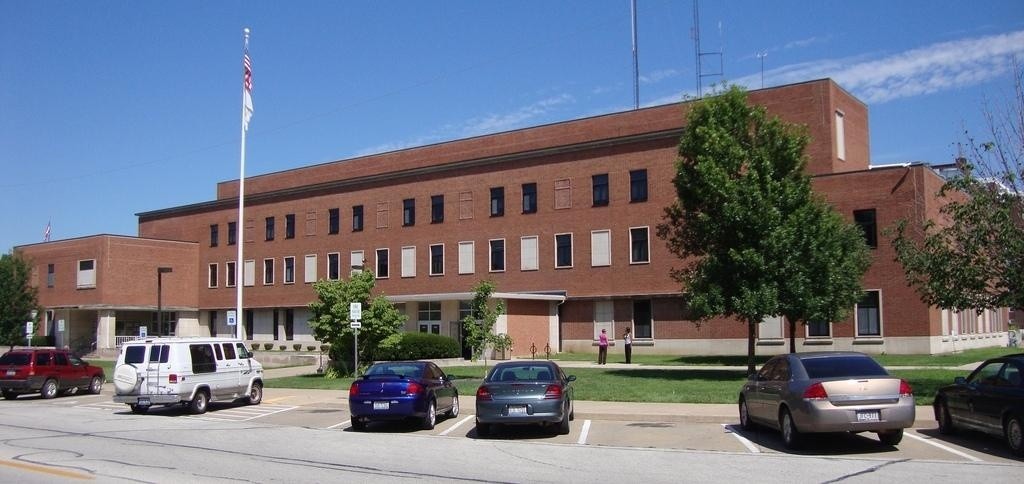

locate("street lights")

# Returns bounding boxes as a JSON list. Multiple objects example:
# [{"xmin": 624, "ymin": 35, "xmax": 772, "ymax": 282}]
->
[{"xmin": 155, "ymin": 266, "xmax": 172, "ymax": 335}]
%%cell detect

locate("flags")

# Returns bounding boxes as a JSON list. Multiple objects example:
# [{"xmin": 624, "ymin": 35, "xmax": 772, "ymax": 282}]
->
[
  {"xmin": 240, "ymin": 40, "xmax": 255, "ymax": 132},
  {"xmin": 44, "ymin": 223, "xmax": 53, "ymax": 241}
]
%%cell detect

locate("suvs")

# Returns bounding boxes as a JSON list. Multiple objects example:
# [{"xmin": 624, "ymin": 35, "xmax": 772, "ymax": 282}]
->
[
  {"xmin": 0, "ymin": 348, "xmax": 103, "ymax": 402},
  {"xmin": 110, "ymin": 335, "xmax": 264, "ymax": 412}
]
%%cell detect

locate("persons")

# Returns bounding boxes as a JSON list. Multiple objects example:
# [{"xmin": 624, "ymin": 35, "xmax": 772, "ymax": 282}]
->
[
  {"xmin": 623, "ymin": 327, "xmax": 633, "ymax": 365},
  {"xmin": 598, "ymin": 329, "xmax": 610, "ymax": 365}
]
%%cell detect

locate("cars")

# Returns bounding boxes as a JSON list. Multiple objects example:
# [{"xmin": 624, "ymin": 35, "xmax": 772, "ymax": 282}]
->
[
  {"xmin": 736, "ymin": 349, "xmax": 916, "ymax": 450},
  {"xmin": 931, "ymin": 352, "xmax": 1022, "ymax": 457},
  {"xmin": 473, "ymin": 359, "xmax": 577, "ymax": 436},
  {"xmin": 348, "ymin": 359, "xmax": 459, "ymax": 429}
]
